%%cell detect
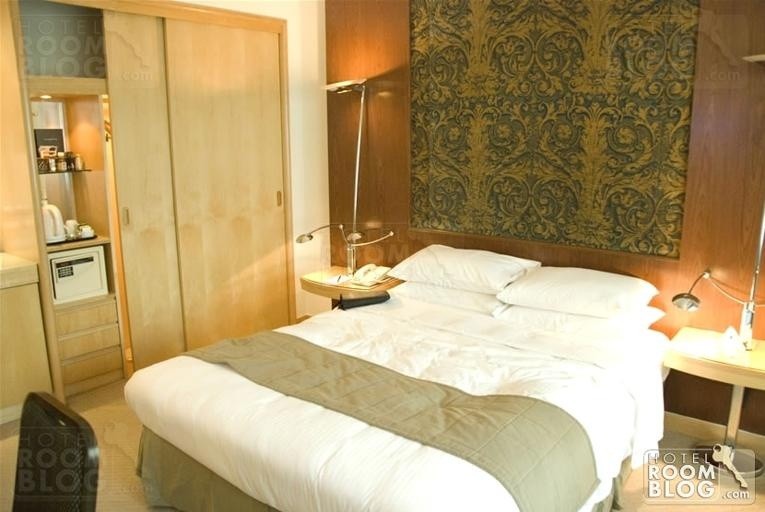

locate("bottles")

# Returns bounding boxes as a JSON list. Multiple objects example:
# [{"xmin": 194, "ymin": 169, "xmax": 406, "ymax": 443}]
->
[{"xmin": 48, "ymin": 149, "xmax": 82, "ymax": 171}]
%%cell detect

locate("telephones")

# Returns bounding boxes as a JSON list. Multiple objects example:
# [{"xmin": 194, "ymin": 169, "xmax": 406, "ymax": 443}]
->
[{"xmin": 351, "ymin": 264, "xmax": 391, "ymax": 286}]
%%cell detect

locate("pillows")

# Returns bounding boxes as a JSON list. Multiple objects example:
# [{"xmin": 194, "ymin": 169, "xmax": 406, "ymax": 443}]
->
[{"xmin": 380, "ymin": 244, "xmax": 667, "ymax": 372}]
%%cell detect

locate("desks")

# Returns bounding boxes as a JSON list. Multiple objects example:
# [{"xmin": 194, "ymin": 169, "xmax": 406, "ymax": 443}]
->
[
  {"xmin": 665, "ymin": 321, "xmax": 765, "ymax": 481},
  {"xmin": 301, "ymin": 261, "xmax": 402, "ymax": 307}
]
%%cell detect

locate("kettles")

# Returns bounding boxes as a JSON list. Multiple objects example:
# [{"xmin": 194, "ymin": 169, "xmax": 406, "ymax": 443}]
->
[{"xmin": 41, "ymin": 204, "xmax": 67, "ymax": 244}]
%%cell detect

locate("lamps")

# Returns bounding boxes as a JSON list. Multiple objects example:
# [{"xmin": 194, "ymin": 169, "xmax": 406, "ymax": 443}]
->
[
  {"xmin": 674, "ymin": 258, "xmax": 760, "ymax": 349},
  {"xmin": 294, "ymin": 70, "xmax": 397, "ymax": 276}
]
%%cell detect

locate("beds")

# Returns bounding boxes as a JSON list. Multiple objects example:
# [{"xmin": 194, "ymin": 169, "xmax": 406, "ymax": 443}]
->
[{"xmin": 112, "ymin": 255, "xmax": 670, "ymax": 511}]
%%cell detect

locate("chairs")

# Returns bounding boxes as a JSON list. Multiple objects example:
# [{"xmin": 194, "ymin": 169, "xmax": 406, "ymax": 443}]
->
[{"xmin": 11, "ymin": 381, "xmax": 100, "ymax": 512}]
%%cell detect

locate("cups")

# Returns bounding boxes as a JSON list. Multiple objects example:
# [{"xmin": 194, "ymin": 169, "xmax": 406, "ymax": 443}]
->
[{"xmin": 65, "ymin": 219, "xmax": 93, "ymax": 238}]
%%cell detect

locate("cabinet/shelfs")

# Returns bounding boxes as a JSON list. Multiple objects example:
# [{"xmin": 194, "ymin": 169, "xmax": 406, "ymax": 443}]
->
[{"xmin": 8, "ymin": 0, "xmax": 285, "ymax": 403}]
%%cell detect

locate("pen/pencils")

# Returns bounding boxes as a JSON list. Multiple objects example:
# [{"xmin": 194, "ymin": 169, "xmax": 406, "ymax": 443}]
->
[{"xmin": 336, "ymin": 274, "xmax": 341, "ymax": 282}]
